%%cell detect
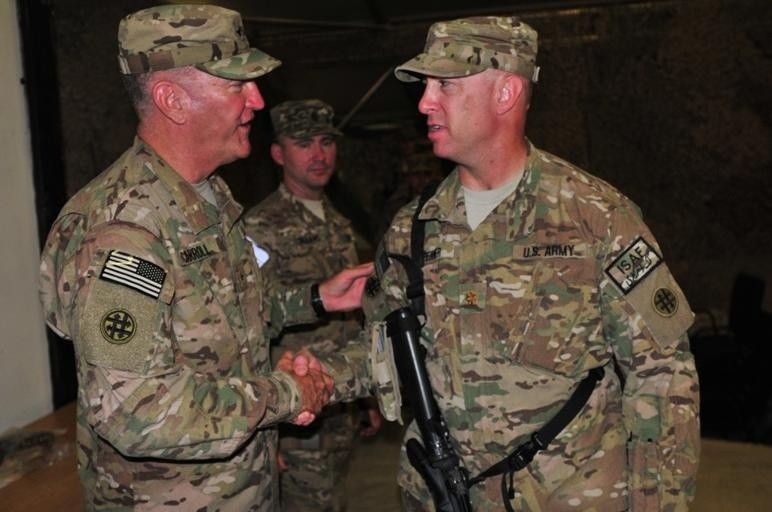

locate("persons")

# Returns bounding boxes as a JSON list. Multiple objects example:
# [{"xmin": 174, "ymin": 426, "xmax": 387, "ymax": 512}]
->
[
  {"xmin": 35, "ymin": 1, "xmax": 373, "ymax": 512},
  {"xmin": 371, "ymin": 137, "xmax": 452, "ymax": 262},
  {"xmin": 243, "ymin": 93, "xmax": 382, "ymax": 511},
  {"xmin": 290, "ymin": 13, "xmax": 704, "ymax": 512}
]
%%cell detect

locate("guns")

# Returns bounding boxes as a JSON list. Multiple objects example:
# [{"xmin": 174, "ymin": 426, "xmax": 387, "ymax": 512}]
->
[{"xmin": 383, "ymin": 305, "xmax": 472, "ymax": 512}]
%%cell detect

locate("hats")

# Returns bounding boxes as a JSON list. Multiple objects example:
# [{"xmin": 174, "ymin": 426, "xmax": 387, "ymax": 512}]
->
[
  {"xmin": 117, "ymin": 3, "xmax": 283, "ymax": 82},
  {"xmin": 393, "ymin": 14, "xmax": 542, "ymax": 85},
  {"xmin": 269, "ymin": 98, "xmax": 344, "ymax": 141}
]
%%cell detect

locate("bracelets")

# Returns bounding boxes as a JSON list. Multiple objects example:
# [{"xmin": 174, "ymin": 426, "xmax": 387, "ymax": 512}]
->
[{"xmin": 311, "ymin": 281, "xmax": 327, "ymax": 317}]
